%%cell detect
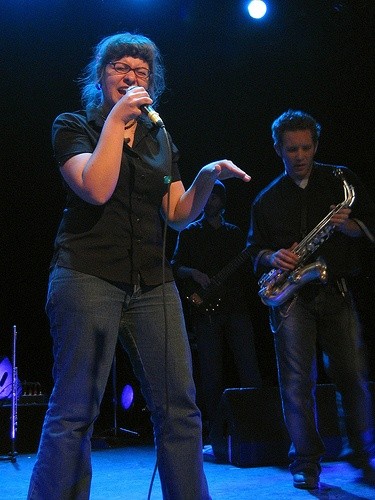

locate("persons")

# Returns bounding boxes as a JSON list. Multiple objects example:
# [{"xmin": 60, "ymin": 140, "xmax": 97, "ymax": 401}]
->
[
  {"xmin": 248, "ymin": 111, "xmax": 375, "ymax": 489},
  {"xmin": 26, "ymin": 34, "xmax": 252, "ymax": 500},
  {"xmin": 170, "ymin": 179, "xmax": 245, "ymax": 439}
]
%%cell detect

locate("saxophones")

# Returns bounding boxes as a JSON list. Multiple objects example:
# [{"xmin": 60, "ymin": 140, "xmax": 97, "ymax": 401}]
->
[{"xmin": 258, "ymin": 167, "xmax": 356, "ymax": 310}]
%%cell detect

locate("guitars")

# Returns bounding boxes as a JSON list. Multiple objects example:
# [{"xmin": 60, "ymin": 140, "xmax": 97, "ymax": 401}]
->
[{"xmin": 182, "ymin": 248, "xmax": 249, "ymax": 315}]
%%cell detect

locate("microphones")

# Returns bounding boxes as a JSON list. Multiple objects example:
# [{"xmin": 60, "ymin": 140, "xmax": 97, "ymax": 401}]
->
[{"xmin": 125, "ymin": 85, "xmax": 165, "ymax": 128}]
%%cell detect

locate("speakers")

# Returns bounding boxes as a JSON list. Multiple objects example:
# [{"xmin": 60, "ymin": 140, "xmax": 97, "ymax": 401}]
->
[
  {"xmin": 0, "ymin": 403, "xmax": 50, "ymax": 453},
  {"xmin": 209, "ymin": 385, "xmax": 294, "ymax": 467},
  {"xmin": 317, "ymin": 383, "xmax": 342, "ymax": 461}
]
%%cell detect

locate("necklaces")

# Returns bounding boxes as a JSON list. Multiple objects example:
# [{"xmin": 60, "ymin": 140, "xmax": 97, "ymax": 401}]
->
[{"xmin": 124, "ymin": 118, "xmax": 137, "ymax": 130}]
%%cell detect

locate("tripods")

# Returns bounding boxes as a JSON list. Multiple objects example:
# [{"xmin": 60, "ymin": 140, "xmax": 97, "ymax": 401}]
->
[{"xmin": 93, "ymin": 352, "xmax": 142, "ymax": 439}]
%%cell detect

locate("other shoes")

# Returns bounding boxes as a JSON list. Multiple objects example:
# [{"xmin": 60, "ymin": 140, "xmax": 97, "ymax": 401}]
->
[
  {"xmin": 363, "ymin": 458, "xmax": 375, "ymax": 477},
  {"xmin": 293, "ymin": 468, "xmax": 320, "ymax": 489}
]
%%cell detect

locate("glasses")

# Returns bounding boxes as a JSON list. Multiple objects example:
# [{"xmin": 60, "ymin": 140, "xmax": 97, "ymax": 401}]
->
[{"xmin": 107, "ymin": 61, "xmax": 152, "ymax": 79}]
